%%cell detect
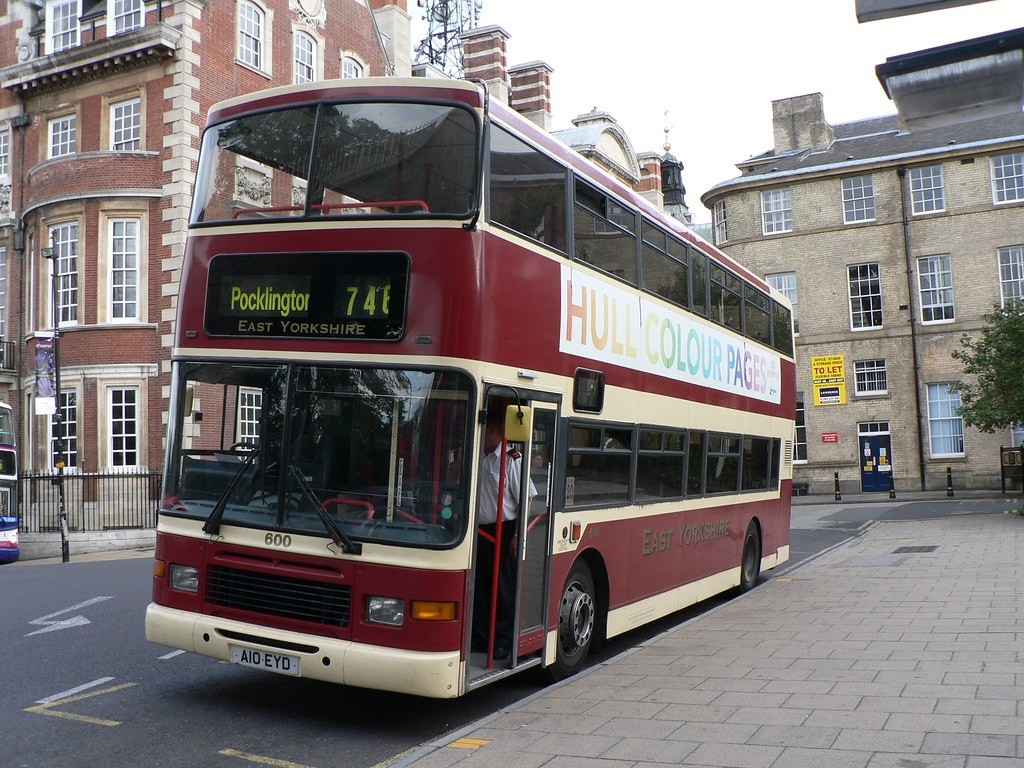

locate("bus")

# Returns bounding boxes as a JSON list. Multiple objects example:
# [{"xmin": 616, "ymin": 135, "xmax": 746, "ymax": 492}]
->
[
  {"xmin": 0, "ymin": 403, "xmax": 20, "ymax": 564},
  {"xmin": 143, "ymin": 77, "xmax": 797, "ymax": 701}
]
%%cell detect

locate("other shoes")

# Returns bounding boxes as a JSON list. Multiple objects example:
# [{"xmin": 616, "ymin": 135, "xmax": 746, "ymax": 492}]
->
[{"xmin": 493, "ymin": 647, "xmax": 509, "ymax": 660}]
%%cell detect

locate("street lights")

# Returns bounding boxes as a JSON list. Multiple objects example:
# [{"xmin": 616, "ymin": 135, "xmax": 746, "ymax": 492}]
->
[{"xmin": 41, "ymin": 228, "xmax": 73, "ymax": 563}]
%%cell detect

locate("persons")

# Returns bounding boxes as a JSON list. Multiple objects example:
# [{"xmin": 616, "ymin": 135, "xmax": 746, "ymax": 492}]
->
[{"xmin": 453, "ymin": 412, "xmax": 538, "ymax": 661}]
